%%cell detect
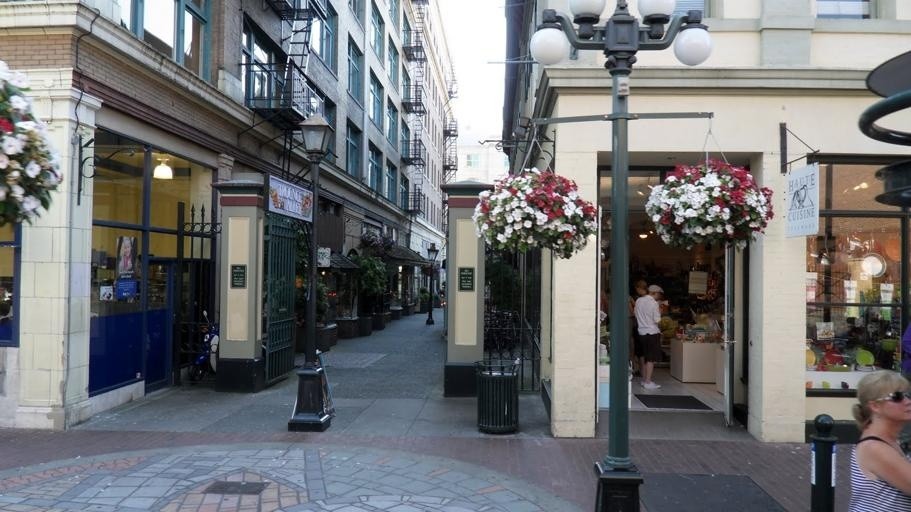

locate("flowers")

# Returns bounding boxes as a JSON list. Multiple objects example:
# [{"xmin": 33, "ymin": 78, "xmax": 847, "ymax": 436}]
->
[
  {"xmin": 643, "ymin": 154, "xmax": 778, "ymax": 252},
  {"xmin": 0, "ymin": 53, "xmax": 65, "ymax": 230},
  {"xmin": 472, "ymin": 164, "xmax": 606, "ymax": 264}
]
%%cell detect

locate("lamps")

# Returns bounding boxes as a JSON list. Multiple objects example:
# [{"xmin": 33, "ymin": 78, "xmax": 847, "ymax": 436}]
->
[{"xmin": 151, "ymin": 157, "xmax": 175, "ymax": 181}]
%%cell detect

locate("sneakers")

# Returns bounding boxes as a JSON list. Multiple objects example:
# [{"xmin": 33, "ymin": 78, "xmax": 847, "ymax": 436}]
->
[{"xmin": 640, "ymin": 381, "xmax": 661, "ymax": 390}]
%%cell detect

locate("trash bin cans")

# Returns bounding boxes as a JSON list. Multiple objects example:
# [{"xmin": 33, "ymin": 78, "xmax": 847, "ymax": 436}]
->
[{"xmin": 475, "ymin": 360, "xmax": 520, "ymax": 434}]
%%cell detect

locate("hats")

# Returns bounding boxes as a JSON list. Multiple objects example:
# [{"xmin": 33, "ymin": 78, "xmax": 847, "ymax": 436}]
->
[{"xmin": 648, "ymin": 284, "xmax": 664, "ymax": 293}]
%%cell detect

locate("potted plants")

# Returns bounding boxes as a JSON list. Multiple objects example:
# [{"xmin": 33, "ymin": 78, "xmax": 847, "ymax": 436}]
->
[{"xmin": 286, "ymin": 228, "xmax": 445, "ymax": 354}]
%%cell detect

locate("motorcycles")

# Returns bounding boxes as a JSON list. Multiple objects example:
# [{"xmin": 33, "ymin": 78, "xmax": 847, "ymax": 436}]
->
[{"xmin": 189, "ymin": 311, "xmax": 269, "ymax": 386}]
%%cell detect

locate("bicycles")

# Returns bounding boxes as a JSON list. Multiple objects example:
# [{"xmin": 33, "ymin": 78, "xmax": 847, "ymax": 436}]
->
[{"xmin": 485, "ymin": 308, "xmax": 521, "ymax": 359}]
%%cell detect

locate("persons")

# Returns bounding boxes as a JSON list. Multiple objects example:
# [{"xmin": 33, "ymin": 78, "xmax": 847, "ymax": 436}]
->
[
  {"xmin": 848, "ymin": 372, "xmax": 911, "ymax": 512},
  {"xmin": 626, "ymin": 279, "xmax": 664, "ymax": 390}
]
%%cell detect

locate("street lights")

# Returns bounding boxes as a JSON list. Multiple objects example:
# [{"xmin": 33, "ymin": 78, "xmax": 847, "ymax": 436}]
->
[
  {"xmin": 291, "ymin": 112, "xmax": 337, "ymax": 432},
  {"xmin": 425, "ymin": 242, "xmax": 442, "ymax": 325},
  {"xmin": 530, "ymin": 0, "xmax": 715, "ymax": 512}
]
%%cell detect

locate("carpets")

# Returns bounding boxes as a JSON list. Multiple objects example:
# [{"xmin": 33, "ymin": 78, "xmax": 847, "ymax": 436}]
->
[
  {"xmin": 635, "ymin": 472, "xmax": 791, "ymax": 511},
  {"xmin": 634, "ymin": 392, "xmax": 715, "ymax": 410}
]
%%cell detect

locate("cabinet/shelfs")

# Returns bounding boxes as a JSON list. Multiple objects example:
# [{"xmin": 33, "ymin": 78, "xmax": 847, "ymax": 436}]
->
[
  {"xmin": 597, "ymin": 358, "xmax": 634, "ymax": 410},
  {"xmin": 664, "ymin": 336, "xmax": 717, "ymax": 384},
  {"xmin": 715, "ymin": 343, "xmax": 726, "ymax": 393},
  {"xmin": 150, "ymin": 263, "xmax": 194, "ymax": 327}
]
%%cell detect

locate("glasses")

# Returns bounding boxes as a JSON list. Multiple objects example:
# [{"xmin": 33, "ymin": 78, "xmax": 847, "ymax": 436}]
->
[{"xmin": 874, "ymin": 392, "xmax": 911, "ymax": 403}]
